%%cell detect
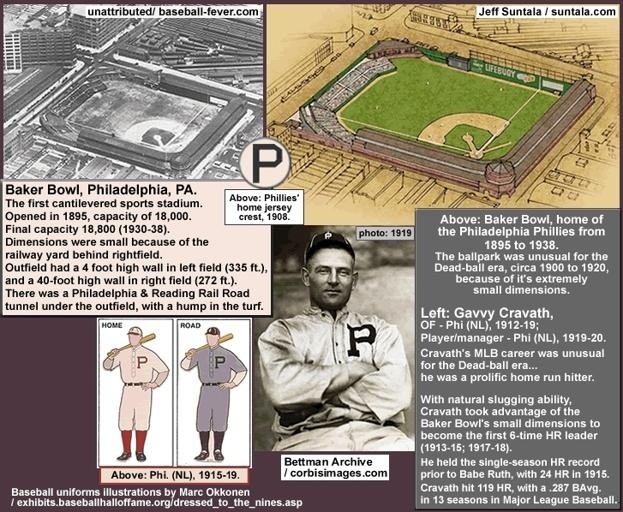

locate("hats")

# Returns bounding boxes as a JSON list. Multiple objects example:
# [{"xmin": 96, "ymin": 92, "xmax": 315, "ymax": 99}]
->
[
  {"xmin": 127, "ymin": 327, "xmax": 141, "ymax": 336},
  {"xmin": 205, "ymin": 328, "xmax": 220, "ymax": 335},
  {"xmin": 303, "ymin": 232, "xmax": 355, "ymax": 267}
]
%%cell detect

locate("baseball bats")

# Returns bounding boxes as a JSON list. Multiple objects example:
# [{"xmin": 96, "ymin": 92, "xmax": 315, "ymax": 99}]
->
[
  {"xmin": 107, "ymin": 334, "xmax": 156, "ymax": 357},
  {"xmin": 186, "ymin": 332, "xmax": 233, "ymax": 357}
]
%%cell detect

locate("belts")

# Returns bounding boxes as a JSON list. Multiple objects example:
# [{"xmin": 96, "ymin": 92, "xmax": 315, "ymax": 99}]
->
[
  {"xmin": 201, "ymin": 383, "xmax": 223, "ymax": 386},
  {"xmin": 124, "ymin": 382, "xmax": 144, "ymax": 387}
]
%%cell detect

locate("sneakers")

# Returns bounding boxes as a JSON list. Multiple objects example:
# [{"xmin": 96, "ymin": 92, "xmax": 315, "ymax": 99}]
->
[
  {"xmin": 213, "ymin": 449, "xmax": 224, "ymax": 461},
  {"xmin": 135, "ymin": 450, "xmax": 146, "ymax": 462},
  {"xmin": 116, "ymin": 452, "xmax": 131, "ymax": 460},
  {"xmin": 195, "ymin": 451, "xmax": 210, "ymax": 461}
]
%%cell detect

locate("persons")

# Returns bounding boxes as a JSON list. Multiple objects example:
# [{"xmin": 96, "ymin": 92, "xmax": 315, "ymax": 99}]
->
[
  {"xmin": 257, "ymin": 231, "xmax": 416, "ymax": 452},
  {"xmin": 181, "ymin": 328, "xmax": 247, "ymax": 461},
  {"xmin": 103, "ymin": 327, "xmax": 169, "ymax": 459}
]
